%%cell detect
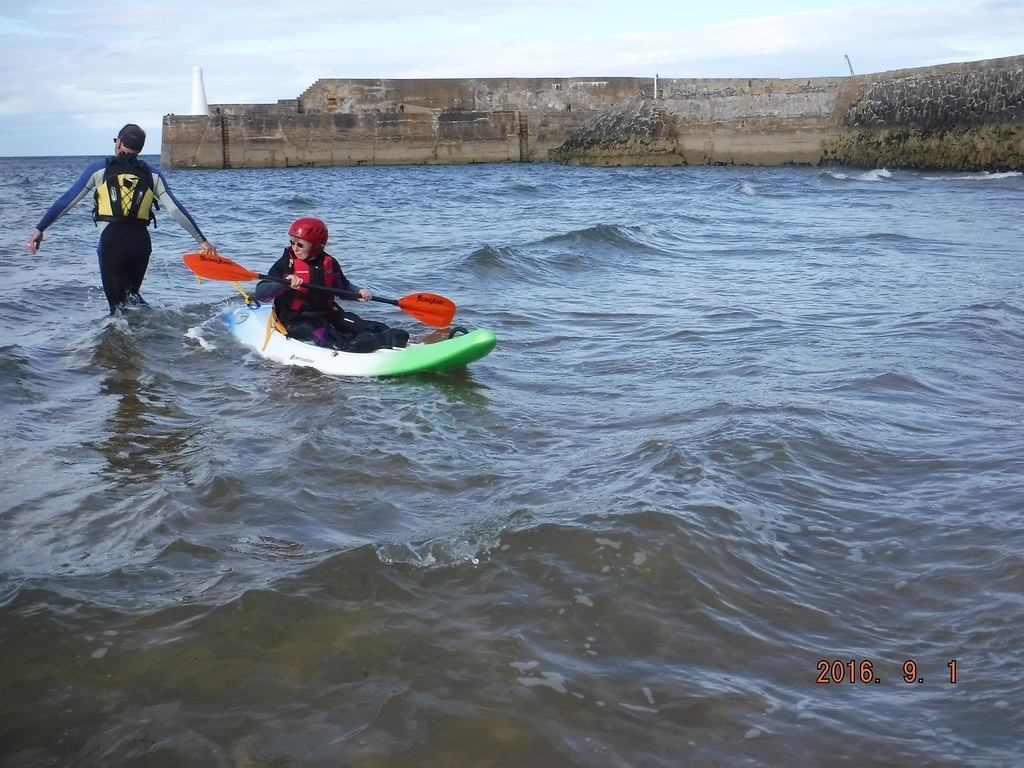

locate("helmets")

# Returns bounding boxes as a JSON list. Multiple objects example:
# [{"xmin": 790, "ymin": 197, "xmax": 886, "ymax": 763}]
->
[{"xmin": 288, "ymin": 216, "xmax": 328, "ymax": 244}]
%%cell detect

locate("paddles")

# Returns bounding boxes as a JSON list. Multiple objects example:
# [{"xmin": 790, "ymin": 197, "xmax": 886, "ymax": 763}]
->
[{"xmin": 180, "ymin": 251, "xmax": 457, "ymax": 329}]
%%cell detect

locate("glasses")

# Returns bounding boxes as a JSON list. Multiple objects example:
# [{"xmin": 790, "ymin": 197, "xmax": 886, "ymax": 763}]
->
[
  {"xmin": 289, "ymin": 240, "xmax": 304, "ymax": 248},
  {"xmin": 113, "ymin": 137, "xmax": 117, "ymax": 143}
]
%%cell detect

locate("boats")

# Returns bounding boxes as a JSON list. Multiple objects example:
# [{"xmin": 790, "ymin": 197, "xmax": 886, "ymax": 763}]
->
[{"xmin": 225, "ymin": 303, "xmax": 500, "ymax": 379}]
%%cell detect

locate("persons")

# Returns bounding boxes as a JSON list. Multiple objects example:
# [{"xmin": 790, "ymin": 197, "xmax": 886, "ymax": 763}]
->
[
  {"xmin": 28, "ymin": 123, "xmax": 218, "ymax": 325},
  {"xmin": 255, "ymin": 217, "xmax": 409, "ymax": 354}
]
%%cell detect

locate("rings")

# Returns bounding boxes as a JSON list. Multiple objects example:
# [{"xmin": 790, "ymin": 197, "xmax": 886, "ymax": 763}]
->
[{"xmin": 29, "ymin": 244, "xmax": 32, "ymax": 246}]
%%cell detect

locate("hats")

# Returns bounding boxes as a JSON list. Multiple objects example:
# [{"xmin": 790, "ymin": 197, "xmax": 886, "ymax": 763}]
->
[{"xmin": 119, "ymin": 123, "xmax": 146, "ymax": 151}]
